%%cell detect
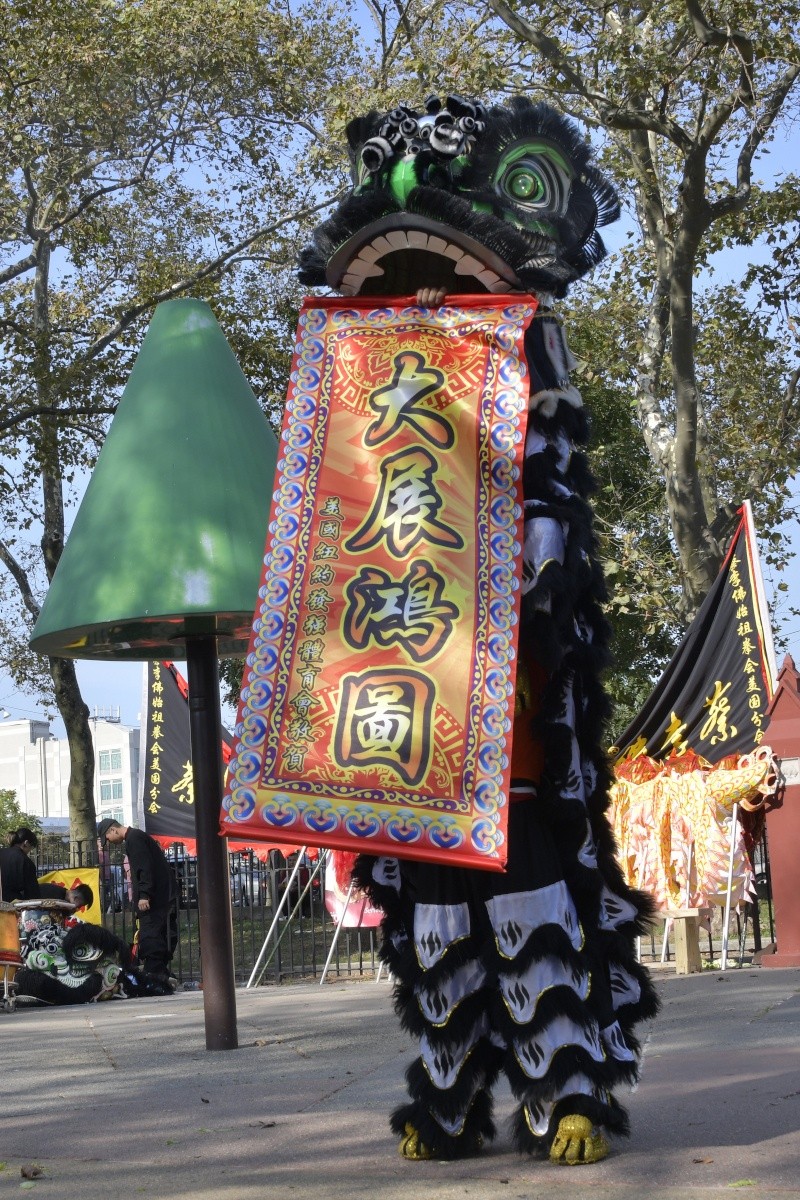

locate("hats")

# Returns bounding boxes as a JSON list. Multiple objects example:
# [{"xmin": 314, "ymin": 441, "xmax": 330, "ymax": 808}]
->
[{"xmin": 97, "ymin": 818, "xmax": 117, "ymax": 847}]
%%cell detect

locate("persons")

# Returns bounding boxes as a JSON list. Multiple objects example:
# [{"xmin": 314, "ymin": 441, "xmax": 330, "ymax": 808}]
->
[
  {"xmin": 278, "ymin": 854, "xmax": 309, "ymax": 918},
  {"xmin": 36, "ymin": 883, "xmax": 94, "ymax": 911},
  {"xmin": 98, "ymin": 817, "xmax": 175, "ymax": 975},
  {"xmin": 0, "ymin": 829, "xmax": 39, "ymax": 902}
]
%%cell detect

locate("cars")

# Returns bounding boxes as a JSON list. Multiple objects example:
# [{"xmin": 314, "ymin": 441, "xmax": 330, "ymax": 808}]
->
[{"xmin": 36, "ymin": 854, "xmax": 322, "ymax": 908}]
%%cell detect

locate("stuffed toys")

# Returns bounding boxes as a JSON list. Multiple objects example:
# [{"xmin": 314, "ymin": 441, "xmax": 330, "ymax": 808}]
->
[{"xmin": 301, "ymin": 91, "xmax": 661, "ymax": 1162}]
